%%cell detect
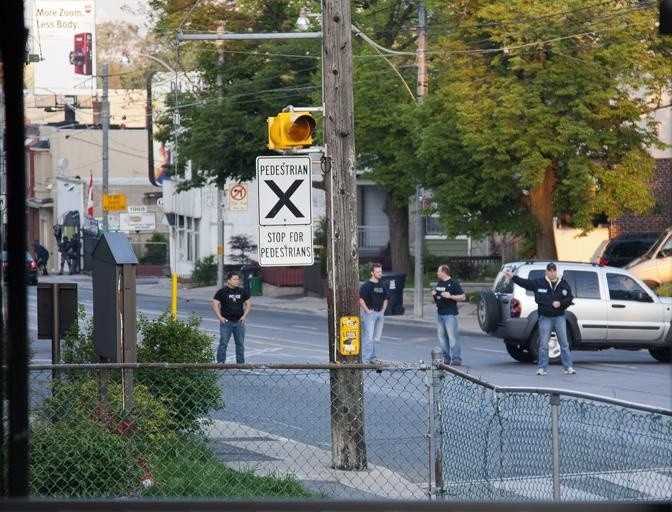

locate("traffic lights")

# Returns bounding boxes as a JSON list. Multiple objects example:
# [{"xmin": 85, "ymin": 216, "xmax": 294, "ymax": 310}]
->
[{"xmin": 274, "ymin": 113, "xmax": 314, "ymax": 147}]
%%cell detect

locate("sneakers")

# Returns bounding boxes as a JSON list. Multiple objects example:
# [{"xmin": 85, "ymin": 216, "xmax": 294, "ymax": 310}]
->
[
  {"xmin": 536, "ymin": 368, "xmax": 547, "ymax": 375},
  {"xmin": 217, "ymin": 361, "xmax": 224, "ymax": 370},
  {"xmin": 444, "ymin": 359, "xmax": 461, "ymax": 366},
  {"xmin": 564, "ymin": 367, "xmax": 576, "ymax": 374}
]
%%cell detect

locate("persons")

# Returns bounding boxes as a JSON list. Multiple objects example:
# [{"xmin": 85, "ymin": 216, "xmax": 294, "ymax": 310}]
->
[
  {"xmin": 29, "ymin": 240, "xmax": 50, "ymax": 276},
  {"xmin": 503, "ymin": 261, "xmax": 579, "ymax": 376},
  {"xmin": 359, "ymin": 262, "xmax": 391, "ymax": 365},
  {"xmin": 432, "ymin": 263, "xmax": 468, "ymax": 367},
  {"xmin": 54, "ymin": 234, "xmax": 72, "ymax": 276},
  {"xmin": 210, "ymin": 271, "xmax": 253, "ymax": 364},
  {"xmin": 66, "ymin": 232, "xmax": 83, "ymax": 275}
]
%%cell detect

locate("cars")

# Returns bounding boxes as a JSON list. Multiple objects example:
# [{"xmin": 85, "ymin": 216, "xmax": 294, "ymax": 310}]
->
[
  {"xmin": 1, "ymin": 250, "xmax": 39, "ymax": 285},
  {"xmin": 599, "ymin": 227, "xmax": 671, "ymax": 294}
]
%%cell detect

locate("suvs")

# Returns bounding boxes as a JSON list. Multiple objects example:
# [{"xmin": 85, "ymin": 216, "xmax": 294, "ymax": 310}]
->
[{"xmin": 478, "ymin": 259, "xmax": 671, "ymax": 363}]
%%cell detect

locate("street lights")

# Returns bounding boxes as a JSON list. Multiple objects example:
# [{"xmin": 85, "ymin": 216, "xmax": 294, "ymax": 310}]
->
[
  {"xmin": 22, "ymin": 62, "xmax": 109, "ymax": 232},
  {"xmin": 127, "ymin": 29, "xmax": 226, "ymax": 291}
]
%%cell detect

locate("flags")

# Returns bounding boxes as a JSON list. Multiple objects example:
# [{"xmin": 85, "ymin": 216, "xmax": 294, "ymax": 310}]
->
[{"xmin": 85, "ymin": 175, "xmax": 97, "ymax": 218}]
